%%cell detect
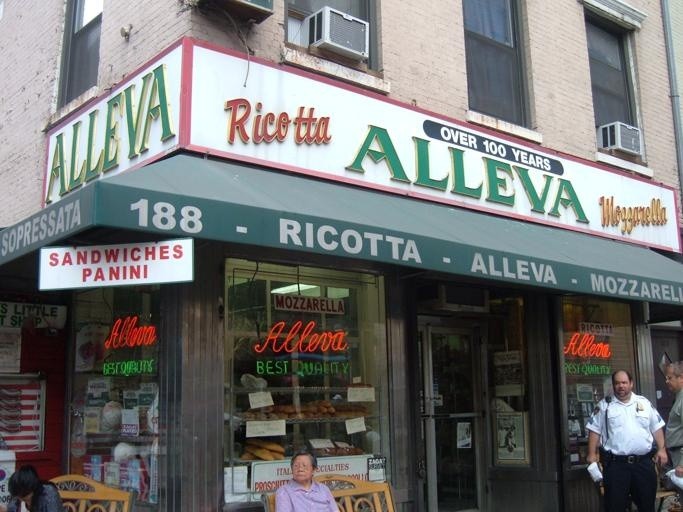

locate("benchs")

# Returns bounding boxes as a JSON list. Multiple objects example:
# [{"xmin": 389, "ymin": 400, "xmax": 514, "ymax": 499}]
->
[
  {"xmin": 45, "ymin": 474, "xmax": 134, "ymax": 512},
  {"xmin": 599, "ymin": 460, "xmax": 676, "ymax": 512},
  {"xmin": 261, "ymin": 473, "xmax": 398, "ymax": 511}
]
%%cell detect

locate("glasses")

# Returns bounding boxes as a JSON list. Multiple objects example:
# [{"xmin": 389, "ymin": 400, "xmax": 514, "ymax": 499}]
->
[{"xmin": 665, "ymin": 375, "xmax": 680, "ymax": 381}]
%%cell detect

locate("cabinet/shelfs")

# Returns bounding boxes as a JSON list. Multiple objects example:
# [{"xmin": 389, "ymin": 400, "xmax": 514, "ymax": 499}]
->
[
  {"xmin": 224, "ymin": 384, "xmax": 380, "ymax": 469},
  {"xmin": 81, "ymin": 370, "xmax": 163, "ymax": 510}
]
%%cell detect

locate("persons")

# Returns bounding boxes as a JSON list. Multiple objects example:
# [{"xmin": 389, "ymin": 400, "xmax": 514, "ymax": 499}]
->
[
  {"xmin": 505, "ymin": 425, "xmax": 518, "ymax": 454},
  {"xmin": 664, "ymin": 360, "xmax": 683, "ymax": 511},
  {"xmin": 3, "ymin": 465, "xmax": 66, "ymax": 512},
  {"xmin": 585, "ymin": 369, "xmax": 666, "ymax": 512},
  {"xmin": 275, "ymin": 451, "xmax": 341, "ymax": 512}
]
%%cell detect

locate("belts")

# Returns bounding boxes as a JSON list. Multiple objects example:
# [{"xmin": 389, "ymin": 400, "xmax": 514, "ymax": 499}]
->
[{"xmin": 612, "ymin": 454, "xmax": 651, "ymax": 464}]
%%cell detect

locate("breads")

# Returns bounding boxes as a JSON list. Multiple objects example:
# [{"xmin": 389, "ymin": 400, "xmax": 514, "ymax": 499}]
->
[
  {"xmin": 235, "ymin": 400, "xmax": 371, "ymax": 421},
  {"xmin": 241, "ymin": 438, "xmax": 285, "ymax": 461}
]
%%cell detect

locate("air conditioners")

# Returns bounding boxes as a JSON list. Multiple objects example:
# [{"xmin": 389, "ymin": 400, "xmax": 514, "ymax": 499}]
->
[
  {"xmin": 301, "ymin": 5, "xmax": 372, "ymax": 64},
  {"xmin": 598, "ymin": 121, "xmax": 644, "ymax": 158}
]
